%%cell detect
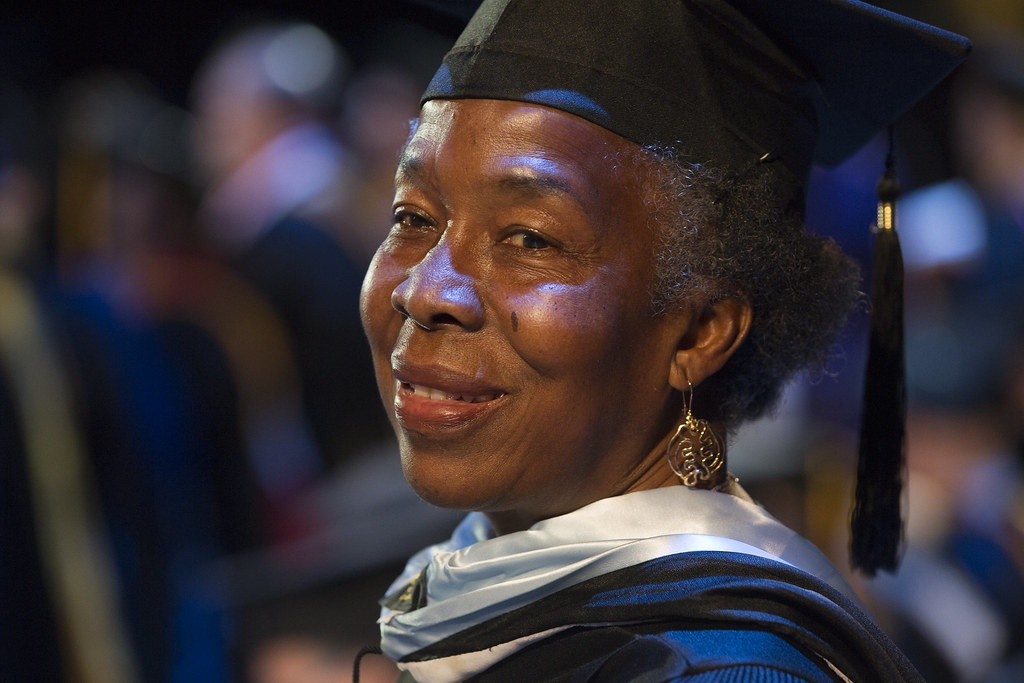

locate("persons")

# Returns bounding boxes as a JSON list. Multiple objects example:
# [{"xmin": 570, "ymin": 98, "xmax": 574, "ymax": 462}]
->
[{"xmin": 353, "ymin": 0, "xmax": 973, "ymax": 683}]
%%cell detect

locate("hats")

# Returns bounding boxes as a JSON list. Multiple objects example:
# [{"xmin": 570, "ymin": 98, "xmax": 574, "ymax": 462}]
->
[{"xmin": 420, "ymin": 0, "xmax": 971, "ymax": 576}]
